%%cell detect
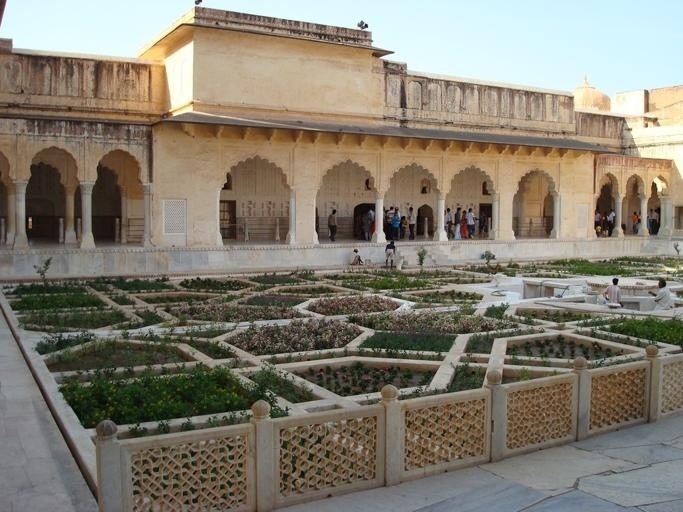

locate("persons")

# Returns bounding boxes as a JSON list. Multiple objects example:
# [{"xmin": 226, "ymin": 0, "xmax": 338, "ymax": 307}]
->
[
  {"xmin": 349, "ymin": 248, "xmax": 363, "ymax": 265},
  {"xmin": 648, "ymin": 279, "xmax": 673, "ymax": 310},
  {"xmin": 384, "ymin": 241, "xmax": 396, "ymax": 270},
  {"xmin": 328, "ymin": 209, "xmax": 338, "ymax": 242},
  {"xmin": 602, "ymin": 278, "xmax": 624, "ymax": 308},
  {"xmin": 442, "ymin": 207, "xmax": 488, "ymax": 239},
  {"xmin": 594, "ymin": 208, "xmax": 660, "ymax": 236},
  {"xmin": 356, "ymin": 203, "xmax": 416, "ymax": 240}
]
